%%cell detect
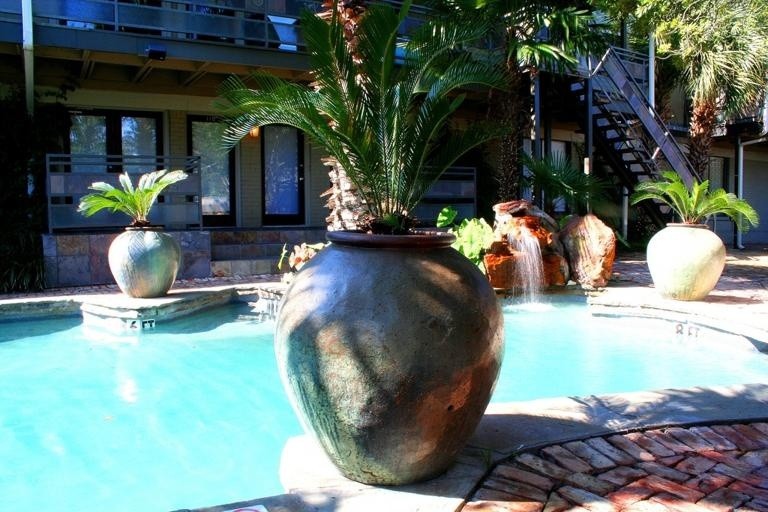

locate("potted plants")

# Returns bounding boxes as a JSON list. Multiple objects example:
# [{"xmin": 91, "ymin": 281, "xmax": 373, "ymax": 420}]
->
[
  {"xmin": 77, "ymin": 170, "xmax": 189, "ymax": 298},
  {"xmin": 219, "ymin": 0, "xmax": 509, "ymax": 489},
  {"xmin": 632, "ymin": 172, "xmax": 759, "ymax": 300}
]
